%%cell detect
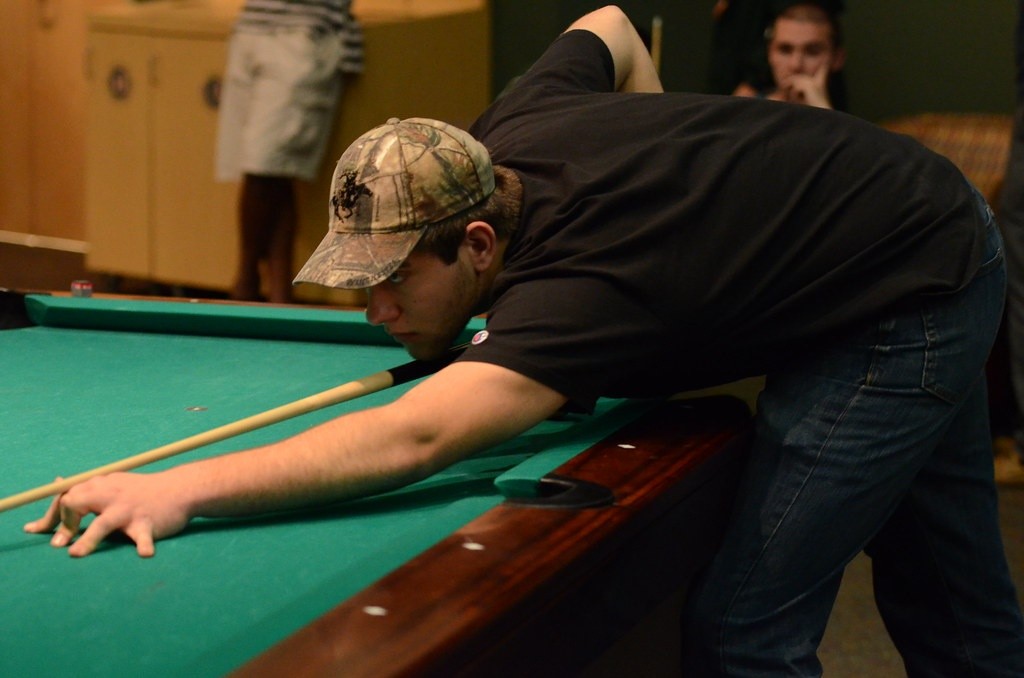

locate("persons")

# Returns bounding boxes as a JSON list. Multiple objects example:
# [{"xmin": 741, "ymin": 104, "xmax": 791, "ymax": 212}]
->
[
  {"xmin": 23, "ymin": 5, "xmax": 1024, "ymax": 678},
  {"xmin": 724, "ymin": 0, "xmax": 848, "ymax": 116},
  {"xmin": 214, "ymin": 0, "xmax": 367, "ymax": 305}
]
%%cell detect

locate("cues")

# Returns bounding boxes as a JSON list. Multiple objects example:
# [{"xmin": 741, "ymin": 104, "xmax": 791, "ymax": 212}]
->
[
  {"xmin": 1, "ymin": 341, "xmax": 473, "ymax": 518},
  {"xmin": 650, "ymin": 14, "xmax": 665, "ymax": 82}
]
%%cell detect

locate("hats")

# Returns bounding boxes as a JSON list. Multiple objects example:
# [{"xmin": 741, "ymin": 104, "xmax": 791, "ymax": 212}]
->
[{"xmin": 291, "ymin": 116, "xmax": 495, "ymax": 290}]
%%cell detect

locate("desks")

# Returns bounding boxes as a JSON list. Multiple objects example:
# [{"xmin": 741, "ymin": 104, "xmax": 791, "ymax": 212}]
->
[{"xmin": 0, "ymin": 284, "xmax": 752, "ymax": 678}]
